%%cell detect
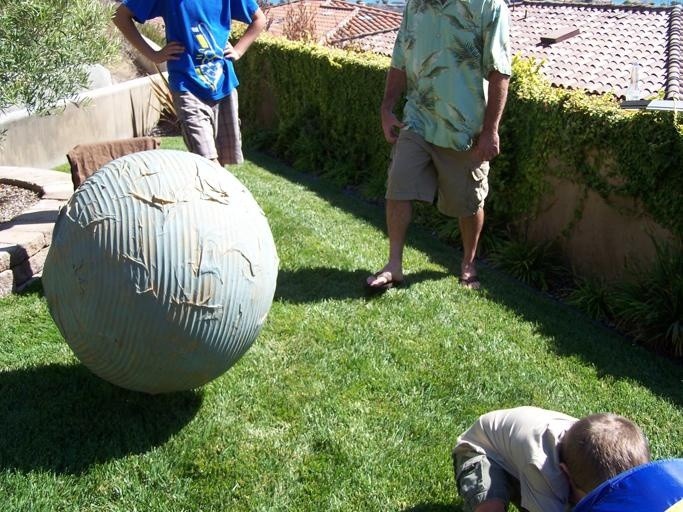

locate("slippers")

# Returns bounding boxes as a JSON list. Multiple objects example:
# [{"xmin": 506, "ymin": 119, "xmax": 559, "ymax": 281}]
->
[
  {"xmin": 459, "ymin": 276, "xmax": 479, "ymax": 290},
  {"xmin": 365, "ymin": 272, "xmax": 406, "ymax": 292}
]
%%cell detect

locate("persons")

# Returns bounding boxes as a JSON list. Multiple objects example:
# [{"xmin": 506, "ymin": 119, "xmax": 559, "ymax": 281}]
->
[
  {"xmin": 113, "ymin": 0, "xmax": 265, "ymax": 169},
  {"xmin": 449, "ymin": 404, "xmax": 649, "ymax": 511},
  {"xmin": 362, "ymin": 0, "xmax": 514, "ymax": 291}
]
